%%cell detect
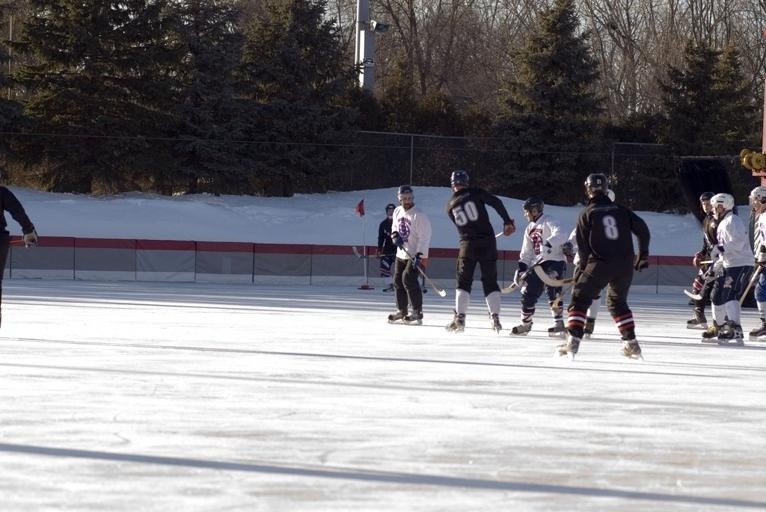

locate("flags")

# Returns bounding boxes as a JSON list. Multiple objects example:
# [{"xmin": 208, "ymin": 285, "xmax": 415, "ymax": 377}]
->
[{"xmin": 356, "ymin": 200, "xmax": 364, "ymax": 218}]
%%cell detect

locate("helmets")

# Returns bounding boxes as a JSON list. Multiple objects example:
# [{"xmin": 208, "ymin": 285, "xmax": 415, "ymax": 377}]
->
[
  {"xmin": 398, "ymin": 186, "xmax": 414, "ymax": 204},
  {"xmin": 701, "ymin": 192, "xmax": 735, "ymax": 222},
  {"xmin": 450, "ymin": 170, "xmax": 469, "ymax": 186},
  {"xmin": 385, "ymin": 204, "xmax": 396, "ymax": 208},
  {"xmin": 584, "ymin": 172, "xmax": 616, "ymax": 203},
  {"xmin": 749, "ymin": 186, "xmax": 766, "ymax": 208},
  {"xmin": 523, "ymin": 196, "xmax": 544, "ymax": 220}
]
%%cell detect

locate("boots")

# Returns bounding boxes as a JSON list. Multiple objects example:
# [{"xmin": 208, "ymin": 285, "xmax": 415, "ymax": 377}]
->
[
  {"xmin": 488, "ymin": 312, "xmax": 502, "ymax": 329},
  {"xmin": 584, "ymin": 319, "xmax": 595, "ymax": 334},
  {"xmin": 512, "ymin": 323, "xmax": 533, "ymax": 333},
  {"xmin": 549, "ymin": 320, "xmax": 565, "ymax": 332},
  {"xmin": 558, "ymin": 329, "xmax": 583, "ymax": 352},
  {"xmin": 446, "ymin": 309, "xmax": 465, "ymax": 329},
  {"xmin": 618, "ymin": 337, "xmax": 642, "ymax": 356}
]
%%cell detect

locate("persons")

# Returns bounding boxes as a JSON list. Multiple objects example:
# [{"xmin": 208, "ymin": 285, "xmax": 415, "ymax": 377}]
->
[
  {"xmin": 512, "ymin": 196, "xmax": 569, "ymax": 336},
  {"xmin": 376, "ymin": 203, "xmax": 396, "ymax": 277},
  {"xmin": 0, "ymin": 184, "xmax": 40, "ymax": 326},
  {"xmin": 684, "ymin": 186, "xmax": 766, "ymax": 340},
  {"xmin": 446, "ymin": 167, "xmax": 515, "ymax": 329},
  {"xmin": 388, "ymin": 184, "xmax": 433, "ymax": 321},
  {"xmin": 557, "ymin": 172, "xmax": 650, "ymax": 356},
  {"xmin": 562, "ymin": 226, "xmax": 602, "ymax": 335}
]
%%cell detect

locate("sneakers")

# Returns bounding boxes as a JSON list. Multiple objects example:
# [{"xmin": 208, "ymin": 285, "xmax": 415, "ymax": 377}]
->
[
  {"xmin": 390, "ymin": 310, "xmax": 408, "ymax": 320},
  {"xmin": 688, "ymin": 307, "xmax": 766, "ymax": 340},
  {"xmin": 404, "ymin": 311, "xmax": 423, "ymax": 321}
]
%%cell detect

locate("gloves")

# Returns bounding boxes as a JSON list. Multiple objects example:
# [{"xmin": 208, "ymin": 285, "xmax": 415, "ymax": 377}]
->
[
  {"xmin": 390, "ymin": 232, "xmax": 403, "ymax": 251},
  {"xmin": 514, "ymin": 269, "xmax": 526, "ymax": 286},
  {"xmin": 634, "ymin": 255, "xmax": 649, "ymax": 271},
  {"xmin": 710, "ymin": 245, "xmax": 720, "ymax": 259},
  {"xmin": 503, "ymin": 221, "xmax": 516, "ymax": 236},
  {"xmin": 412, "ymin": 255, "xmax": 421, "ymax": 271},
  {"xmin": 540, "ymin": 243, "xmax": 552, "ymax": 257},
  {"xmin": 376, "ymin": 248, "xmax": 383, "ymax": 255},
  {"xmin": 692, "ymin": 252, "xmax": 706, "ymax": 268}
]
